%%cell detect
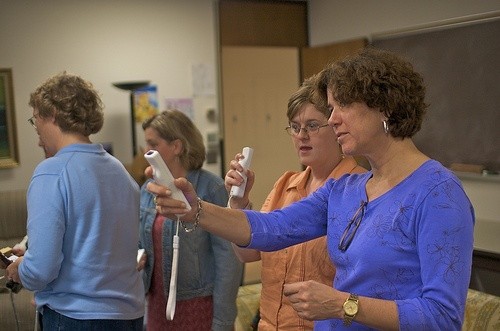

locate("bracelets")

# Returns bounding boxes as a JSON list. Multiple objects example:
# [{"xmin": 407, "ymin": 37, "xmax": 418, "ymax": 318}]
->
[{"xmin": 179, "ymin": 197, "xmax": 202, "ymax": 233}]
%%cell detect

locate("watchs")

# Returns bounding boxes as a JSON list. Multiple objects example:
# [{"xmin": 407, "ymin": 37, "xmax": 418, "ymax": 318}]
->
[{"xmin": 342, "ymin": 292, "xmax": 359, "ymax": 328}]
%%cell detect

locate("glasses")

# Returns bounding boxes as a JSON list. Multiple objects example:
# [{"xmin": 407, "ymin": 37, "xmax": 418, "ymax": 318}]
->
[
  {"xmin": 28, "ymin": 113, "xmax": 40, "ymax": 127},
  {"xmin": 286, "ymin": 122, "xmax": 331, "ymax": 134}
]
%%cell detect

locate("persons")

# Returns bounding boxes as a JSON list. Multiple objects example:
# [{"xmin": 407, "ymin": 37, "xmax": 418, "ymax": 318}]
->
[
  {"xmin": 136, "ymin": 110, "xmax": 243, "ymax": 331},
  {"xmin": 5, "ymin": 74, "xmax": 145, "ymax": 331},
  {"xmin": 144, "ymin": 49, "xmax": 475, "ymax": 331},
  {"xmin": 224, "ymin": 76, "xmax": 369, "ymax": 331}
]
